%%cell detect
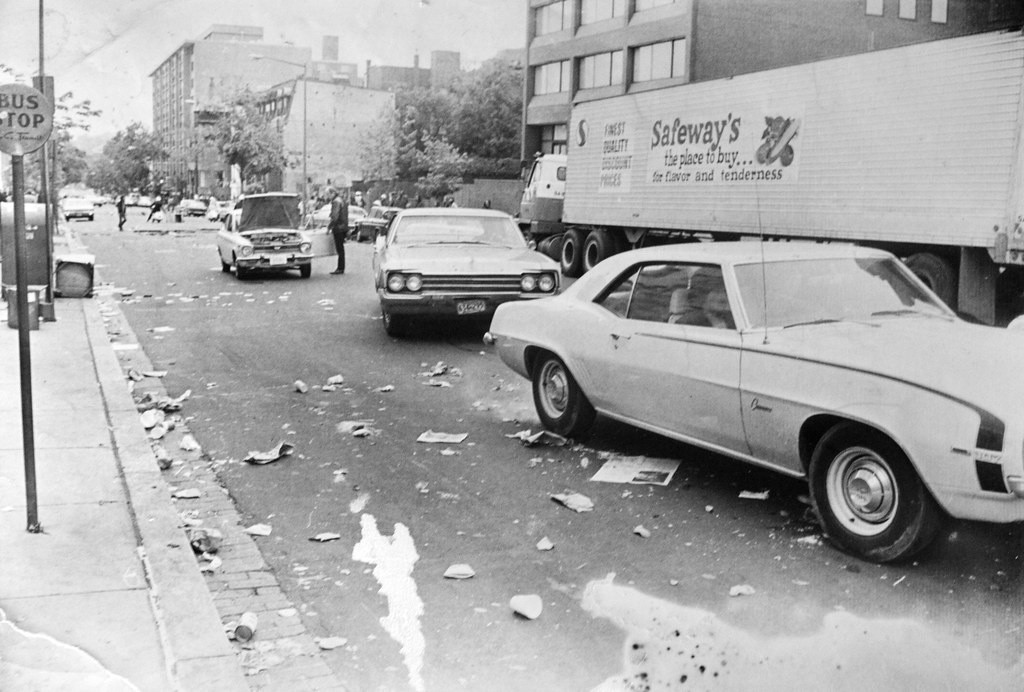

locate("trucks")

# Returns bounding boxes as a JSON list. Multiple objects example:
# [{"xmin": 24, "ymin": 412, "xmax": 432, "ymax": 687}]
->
[{"xmin": 514, "ymin": 27, "xmax": 1024, "ymax": 328}]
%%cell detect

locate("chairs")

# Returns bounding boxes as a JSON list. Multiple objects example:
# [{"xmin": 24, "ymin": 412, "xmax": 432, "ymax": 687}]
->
[{"xmin": 668, "ymin": 287, "xmax": 695, "ymax": 327}]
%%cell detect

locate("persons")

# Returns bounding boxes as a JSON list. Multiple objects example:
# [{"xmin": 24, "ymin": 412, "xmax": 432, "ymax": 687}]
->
[
  {"xmin": 297, "ymin": 190, "xmax": 493, "ymax": 227},
  {"xmin": 674, "ymin": 267, "xmax": 736, "ymax": 328},
  {"xmin": 326, "ymin": 186, "xmax": 348, "ymax": 275},
  {"xmin": 147, "ymin": 196, "xmax": 163, "ymax": 222},
  {"xmin": 0, "ymin": 191, "xmax": 34, "ymax": 202},
  {"xmin": 116, "ymin": 196, "xmax": 127, "ymax": 231}
]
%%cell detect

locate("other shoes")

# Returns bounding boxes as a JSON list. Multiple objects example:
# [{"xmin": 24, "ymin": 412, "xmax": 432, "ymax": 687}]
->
[{"xmin": 330, "ymin": 269, "xmax": 344, "ymax": 274}]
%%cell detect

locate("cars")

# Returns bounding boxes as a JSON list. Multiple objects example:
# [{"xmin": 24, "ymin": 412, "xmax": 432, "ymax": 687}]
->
[
  {"xmin": 62, "ymin": 198, "xmax": 95, "ymax": 221},
  {"xmin": 214, "ymin": 191, "xmax": 314, "ymax": 280},
  {"xmin": 305, "ymin": 203, "xmax": 401, "ymax": 241},
  {"xmin": 125, "ymin": 194, "xmax": 153, "ymax": 206},
  {"xmin": 177, "ymin": 198, "xmax": 235, "ymax": 222},
  {"xmin": 374, "ymin": 207, "xmax": 561, "ymax": 338},
  {"xmin": 489, "ymin": 240, "xmax": 1024, "ymax": 562}
]
